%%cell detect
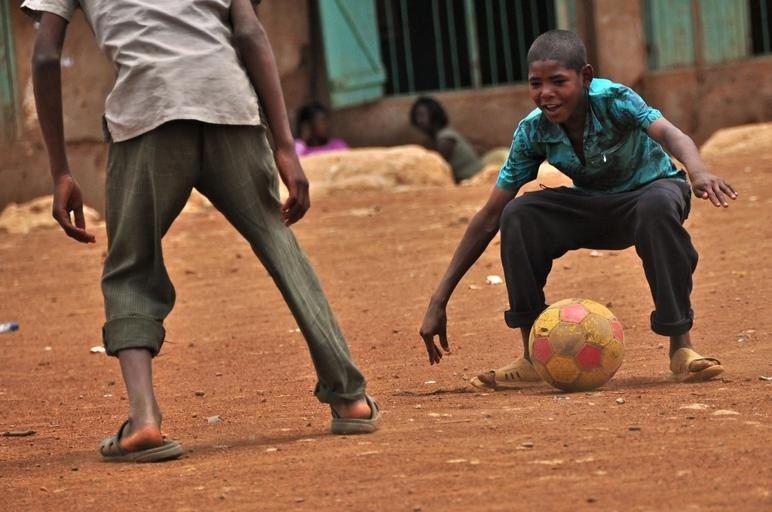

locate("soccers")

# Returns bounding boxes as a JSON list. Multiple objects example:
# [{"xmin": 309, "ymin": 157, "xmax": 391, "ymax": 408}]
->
[{"xmin": 529, "ymin": 298, "xmax": 626, "ymax": 392}]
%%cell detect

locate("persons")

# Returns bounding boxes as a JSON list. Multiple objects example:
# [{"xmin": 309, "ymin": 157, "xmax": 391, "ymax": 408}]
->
[
  {"xmin": 290, "ymin": 102, "xmax": 347, "ymax": 156},
  {"xmin": 20, "ymin": 0, "xmax": 383, "ymax": 462},
  {"xmin": 418, "ymin": 30, "xmax": 743, "ymax": 389},
  {"xmin": 409, "ymin": 96, "xmax": 483, "ymax": 186}
]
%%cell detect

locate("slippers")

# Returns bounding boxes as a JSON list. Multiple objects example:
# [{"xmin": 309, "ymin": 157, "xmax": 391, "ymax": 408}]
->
[
  {"xmin": 99, "ymin": 420, "xmax": 184, "ymax": 461},
  {"xmin": 330, "ymin": 394, "xmax": 381, "ymax": 433},
  {"xmin": 670, "ymin": 347, "xmax": 724, "ymax": 382},
  {"xmin": 470, "ymin": 357, "xmax": 546, "ymax": 391}
]
